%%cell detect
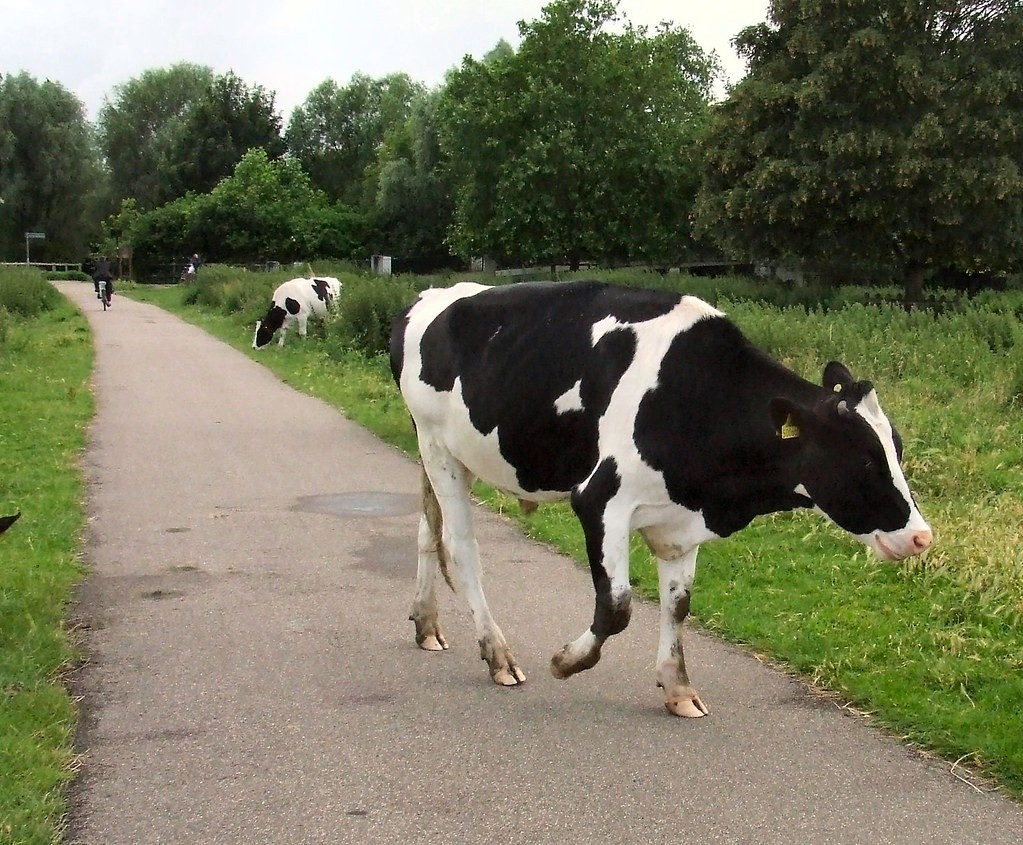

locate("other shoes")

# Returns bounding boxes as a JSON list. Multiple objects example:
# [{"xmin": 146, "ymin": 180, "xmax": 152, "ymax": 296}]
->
[
  {"xmin": 97, "ymin": 294, "xmax": 100, "ymax": 298},
  {"xmin": 107, "ymin": 302, "xmax": 111, "ymax": 307}
]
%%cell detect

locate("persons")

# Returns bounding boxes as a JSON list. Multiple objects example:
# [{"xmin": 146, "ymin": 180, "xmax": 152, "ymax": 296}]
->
[
  {"xmin": 93, "ymin": 256, "xmax": 113, "ymax": 307},
  {"xmin": 190, "ymin": 253, "xmax": 202, "ymax": 272}
]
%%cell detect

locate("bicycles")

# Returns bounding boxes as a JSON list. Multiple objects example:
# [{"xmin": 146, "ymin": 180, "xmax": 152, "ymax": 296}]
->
[{"xmin": 94, "ymin": 278, "xmax": 109, "ymax": 311}]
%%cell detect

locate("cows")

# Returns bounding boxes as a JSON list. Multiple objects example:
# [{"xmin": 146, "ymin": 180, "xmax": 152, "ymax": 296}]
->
[
  {"xmin": 386, "ymin": 279, "xmax": 933, "ymax": 721},
  {"xmin": 250, "ymin": 275, "xmax": 343, "ymax": 352}
]
